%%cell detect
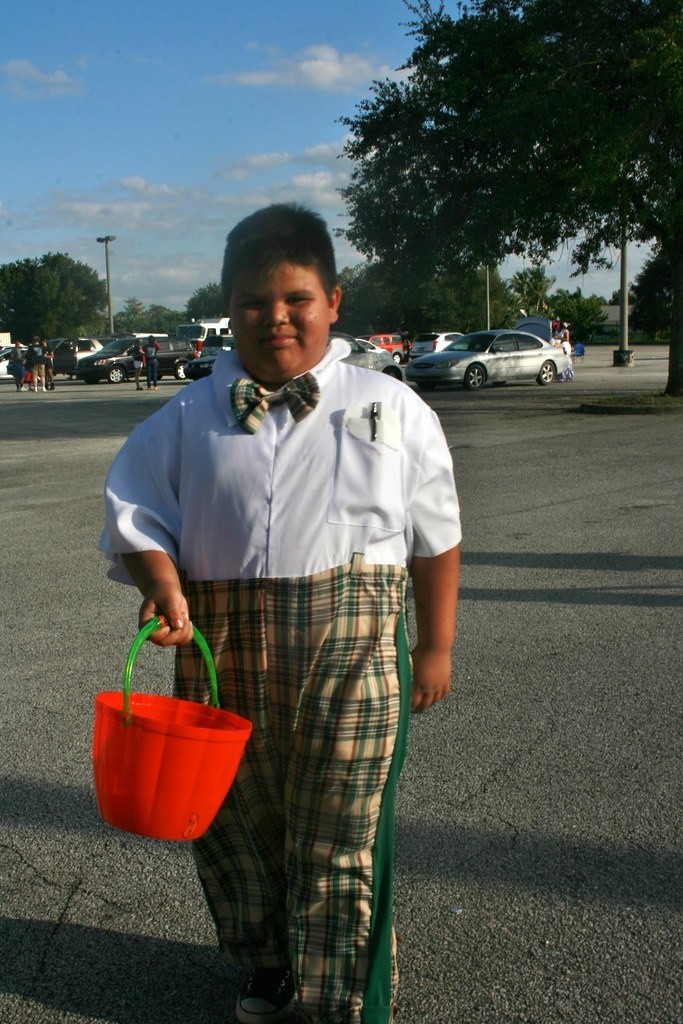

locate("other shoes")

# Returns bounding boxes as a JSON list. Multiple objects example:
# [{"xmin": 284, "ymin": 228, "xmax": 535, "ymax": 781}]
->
[
  {"xmin": 147, "ymin": 387, "xmax": 152, "ymax": 390},
  {"xmin": 16, "ymin": 386, "xmax": 27, "ymax": 392},
  {"xmin": 33, "ymin": 388, "xmax": 38, "ymax": 392},
  {"xmin": 45, "ymin": 383, "xmax": 48, "ymax": 389},
  {"xmin": 136, "ymin": 387, "xmax": 143, "ymax": 390},
  {"xmin": 42, "ymin": 388, "xmax": 47, "ymax": 391},
  {"xmin": 50, "ymin": 382, "xmax": 55, "ymax": 390},
  {"xmin": 154, "ymin": 386, "xmax": 159, "ymax": 390},
  {"xmin": 29, "ymin": 386, "xmax": 34, "ymax": 390}
]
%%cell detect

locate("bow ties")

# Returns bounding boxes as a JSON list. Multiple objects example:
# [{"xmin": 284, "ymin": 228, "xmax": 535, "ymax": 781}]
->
[{"xmin": 229, "ymin": 372, "xmax": 322, "ymax": 435}]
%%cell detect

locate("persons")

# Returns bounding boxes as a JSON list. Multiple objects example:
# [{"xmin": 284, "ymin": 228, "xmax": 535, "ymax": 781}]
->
[
  {"xmin": 6, "ymin": 339, "xmax": 55, "ymax": 392},
  {"xmin": 97, "ymin": 200, "xmax": 463, "ymax": 1024},
  {"xmin": 553, "ymin": 321, "xmax": 575, "ymax": 383},
  {"xmin": 132, "ymin": 335, "xmax": 161, "ymax": 390}
]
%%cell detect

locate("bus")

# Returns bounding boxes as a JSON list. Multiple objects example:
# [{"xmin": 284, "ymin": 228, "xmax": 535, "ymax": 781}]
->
[{"xmin": 176, "ymin": 317, "xmax": 234, "ymax": 358}]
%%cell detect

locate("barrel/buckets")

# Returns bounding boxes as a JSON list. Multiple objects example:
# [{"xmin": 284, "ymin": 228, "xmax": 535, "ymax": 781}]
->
[{"xmin": 92, "ymin": 615, "xmax": 252, "ymax": 841}]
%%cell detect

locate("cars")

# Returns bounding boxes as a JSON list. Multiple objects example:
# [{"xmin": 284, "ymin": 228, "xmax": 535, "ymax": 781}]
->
[
  {"xmin": 405, "ymin": 317, "xmax": 570, "ymax": 391},
  {"xmin": 0, "ymin": 333, "xmax": 405, "ymax": 384}
]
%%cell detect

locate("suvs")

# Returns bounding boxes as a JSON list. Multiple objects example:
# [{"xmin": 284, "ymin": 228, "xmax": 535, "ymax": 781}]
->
[{"xmin": 355, "ymin": 332, "xmax": 465, "ymax": 364}]
